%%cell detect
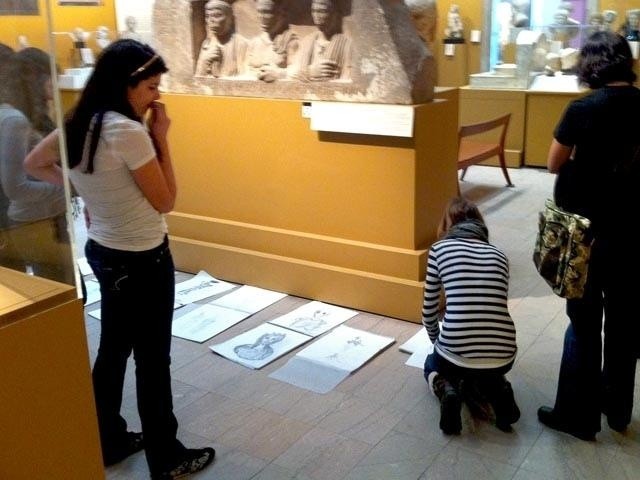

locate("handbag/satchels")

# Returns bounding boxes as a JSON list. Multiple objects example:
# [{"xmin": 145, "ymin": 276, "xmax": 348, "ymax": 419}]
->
[{"xmin": 533, "ymin": 199, "xmax": 595, "ymax": 299}]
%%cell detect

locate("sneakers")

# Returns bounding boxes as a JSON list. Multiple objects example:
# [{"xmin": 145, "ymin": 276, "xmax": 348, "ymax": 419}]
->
[
  {"xmin": 436, "ymin": 379, "xmax": 463, "ymax": 435},
  {"xmin": 150, "ymin": 448, "xmax": 215, "ymax": 480},
  {"xmin": 101, "ymin": 431, "xmax": 145, "ymax": 468},
  {"xmin": 490, "ymin": 380, "xmax": 519, "ymax": 424}
]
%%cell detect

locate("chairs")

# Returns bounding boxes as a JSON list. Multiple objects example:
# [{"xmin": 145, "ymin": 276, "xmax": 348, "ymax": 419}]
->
[{"xmin": 458, "ymin": 113, "xmax": 514, "ymax": 202}]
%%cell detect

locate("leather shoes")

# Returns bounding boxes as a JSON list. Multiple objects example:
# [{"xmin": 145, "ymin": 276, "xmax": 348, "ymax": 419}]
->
[{"xmin": 539, "ymin": 406, "xmax": 627, "ymax": 442}]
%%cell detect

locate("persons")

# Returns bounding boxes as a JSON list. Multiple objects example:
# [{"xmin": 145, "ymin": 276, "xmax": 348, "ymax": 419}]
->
[
  {"xmin": 442, "ymin": 3, "xmax": 466, "ymax": 39},
  {"xmin": 418, "ymin": 196, "xmax": 523, "ymax": 439},
  {"xmin": 20, "ymin": 34, "xmax": 218, "ymax": 478},
  {"xmin": 295, "ymin": 0, "xmax": 349, "ymax": 84},
  {"xmin": 194, "ymin": 0, "xmax": 248, "ymax": 79},
  {"xmin": 243, "ymin": 0, "xmax": 306, "ymax": 83},
  {"xmin": 121, "ymin": 15, "xmax": 143, "ymax": 42},
  {"xmin": 548, "ymin": 3, "xmax": 640, "ymax": 49},
  {"xmin": 1, "ymin": 43, "xmax": 28, "ymax": 276},
  {"xmin": 96, "ymin": 27, "xmax": 112, "ymax": 49},
  {"xmin": 0, "ymin": 48, "xmax": 88, "ymax": 307},
  {"xmin": 536, "ymin": 29, "xmax": 640, "ymax": 442},
  {"xmin": 73, "ymin": 27, "xmax": 84, "ymax": 41}
]
